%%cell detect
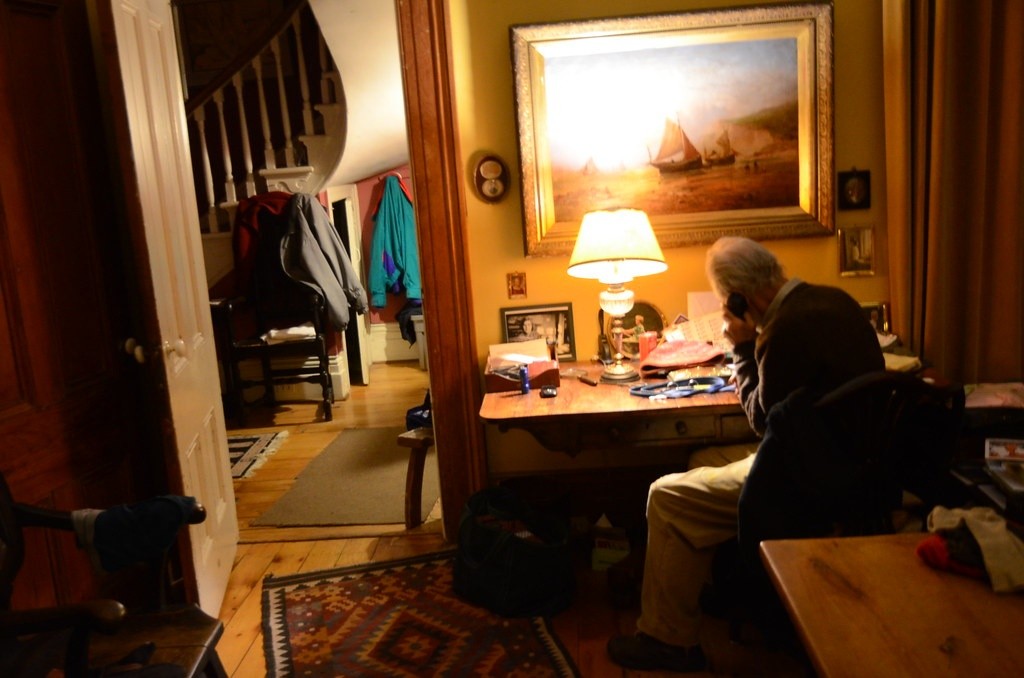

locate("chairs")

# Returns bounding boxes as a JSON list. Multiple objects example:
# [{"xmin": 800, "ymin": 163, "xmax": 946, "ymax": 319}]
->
[
  {"xmin": 210, "ymin": 199, "xmax": 348, "ymax": 421},
  {"xmin": 0, "ymin": 480, "xmax": 228, "ymax": 678}
]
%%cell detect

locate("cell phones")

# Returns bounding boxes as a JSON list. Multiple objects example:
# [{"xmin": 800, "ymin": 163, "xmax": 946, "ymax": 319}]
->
[{"xmin": 542, "ymin": 385, "xmax": 556, "ymax": 397}]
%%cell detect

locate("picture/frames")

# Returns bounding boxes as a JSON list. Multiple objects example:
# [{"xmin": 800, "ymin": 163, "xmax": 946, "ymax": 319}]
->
[
  {"xmin": 860, "ymin": 302, "xmax": 891, "ymax": 330},
  {"xmin": 606, "ymin": 299, "xmax": 667, "ymax": 360},
  {"xmin": 500, "ymin": 304, "xmax": 577, "ymax": 365},
  {"xmin": 837, "ymin": 168, "xmax": 871, "ymax": 208},
  {"xmin": 838, "ymin": 224, "xmax": 877, "ymax": 279},
  {"xmin": 500, "ymin": 6, "xmax": 837, "ymax": 258}
]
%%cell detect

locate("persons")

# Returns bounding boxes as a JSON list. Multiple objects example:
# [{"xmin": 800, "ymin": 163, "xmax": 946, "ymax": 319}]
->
[
  {"xmin": 516, "ymin": 318, "xmax": 541, "ymax": 341},
  {"xmin": 607, "ymin": 236, "xmax": 900, "ymax": 672},
  {"xmin": 868, "ymin": 310, "xmax": 882, "ymax": 330},
  {"xmin": 852, "ymin": 239, "xmax": 864, "ymax": 264},
  {"xmin": 624, "ymin": 314, "xmax": 645, "ymax": 351},
  {"xmin": 490, "ymin": 182, "xmax": 496, "ymax": 193}
]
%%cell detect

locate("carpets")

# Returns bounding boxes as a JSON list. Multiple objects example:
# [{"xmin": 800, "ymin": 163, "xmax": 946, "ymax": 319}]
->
[
  {"xmin": 228, "ymin": 429, "xmax": 290, "ymax": 480},
  {"xmin": 260, "ymin": 547, "xmax": 586, "ymax": 678},
  {"xmin": 252, "ymin": 428, "xmax": 439, "ymax": 527}
]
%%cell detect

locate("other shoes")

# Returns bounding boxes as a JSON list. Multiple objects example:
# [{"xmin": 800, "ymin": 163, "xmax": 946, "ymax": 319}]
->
[
  {"xmin": 702, "ymin": 584, "xmax": 750, "ymax": 623},
  {"xmin": 608, "ymin": 631, "xmax": 703, "ymax": 674}
]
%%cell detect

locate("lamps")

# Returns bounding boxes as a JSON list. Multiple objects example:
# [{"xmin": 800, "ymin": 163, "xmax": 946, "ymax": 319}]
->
[{"xmin": 566, "ymin": 209, "xmax": 669, "ymax": 385}]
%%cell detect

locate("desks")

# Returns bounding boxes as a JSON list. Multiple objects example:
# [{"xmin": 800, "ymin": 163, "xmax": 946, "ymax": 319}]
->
[
  {"xmin": 480, "ymin": 332, "xmax": 916, "ymax": 472},
  {"xmin": 759, "ymin": 530, "xmax": 1024, "ymax": 678}
]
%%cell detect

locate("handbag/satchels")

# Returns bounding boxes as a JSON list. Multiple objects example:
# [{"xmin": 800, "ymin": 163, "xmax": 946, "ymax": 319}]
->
[{"xmin": 451, "ymin": 485, "xmax": 594, "ymax": 618}]
